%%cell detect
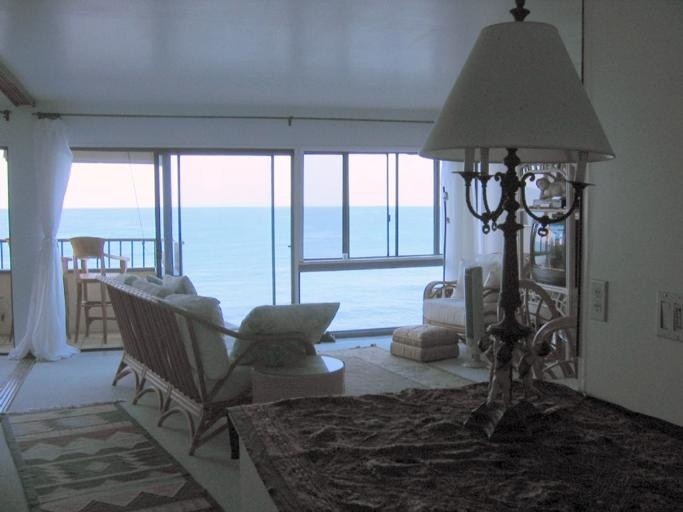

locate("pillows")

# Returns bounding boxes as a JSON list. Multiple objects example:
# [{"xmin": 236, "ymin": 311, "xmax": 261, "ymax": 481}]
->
[
  {"xmin": 452, "ymin": 254, "xmax": 497, "ymax": 300},
  {"xmin": 124, "ymin": 272, "xmax": 342, "ymax": 379}
]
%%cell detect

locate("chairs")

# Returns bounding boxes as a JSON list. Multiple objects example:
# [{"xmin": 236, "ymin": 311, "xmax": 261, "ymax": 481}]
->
[
  {"xmin": 422, "ymin": 251, "xmax": 503, "ymax": 347},
  {"xmin": 70, "ymin": 236, "xmax": 130, "ymax": 344}
]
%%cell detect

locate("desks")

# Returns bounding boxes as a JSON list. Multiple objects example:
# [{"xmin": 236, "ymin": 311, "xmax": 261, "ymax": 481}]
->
[{"xmin": 230, "ymin": 373, "xmax": 683, "ymax": 510}]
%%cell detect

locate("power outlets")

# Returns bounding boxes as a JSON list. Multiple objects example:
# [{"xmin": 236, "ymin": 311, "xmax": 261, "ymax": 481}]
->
[{"xmin": 590, "ymin": 278, "xmax": 609, "ymax": 324}]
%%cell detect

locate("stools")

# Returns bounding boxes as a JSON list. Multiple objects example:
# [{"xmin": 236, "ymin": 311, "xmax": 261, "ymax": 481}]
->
[{"xmin": 389, "ymin": 323, "xmax": 460, "ymax": 363}]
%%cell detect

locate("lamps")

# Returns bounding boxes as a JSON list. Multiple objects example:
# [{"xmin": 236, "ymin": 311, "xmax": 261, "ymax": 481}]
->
[{"xmin": 416, "ymin": 1, "xmax": 616, "ymax": 443}]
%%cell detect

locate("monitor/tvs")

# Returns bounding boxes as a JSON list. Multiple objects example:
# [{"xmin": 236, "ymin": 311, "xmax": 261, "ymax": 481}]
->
[{"xmin": 529, "ymin": 216, "xmax": 581, "ymax": 289}]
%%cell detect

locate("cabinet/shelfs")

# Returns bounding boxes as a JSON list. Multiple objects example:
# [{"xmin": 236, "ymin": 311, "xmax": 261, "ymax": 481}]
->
[{"xmin": 513, "ymin": 163, "xmax": 579, "ymax": 377}]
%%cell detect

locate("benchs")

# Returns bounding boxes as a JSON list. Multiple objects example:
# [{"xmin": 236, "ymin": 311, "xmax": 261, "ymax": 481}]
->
[{"xmin": 92, "ymin": 273, "xmax": 348, "ymax": 457}]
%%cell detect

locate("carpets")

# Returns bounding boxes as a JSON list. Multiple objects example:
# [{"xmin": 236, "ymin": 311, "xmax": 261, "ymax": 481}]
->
[
  {"xmin": 318, "ymin": 344, "xmax": 479, "ymax": 396},
  {"xmin": 0, "ymin": 400, "xmax": 223, "ymax": 510}
]
